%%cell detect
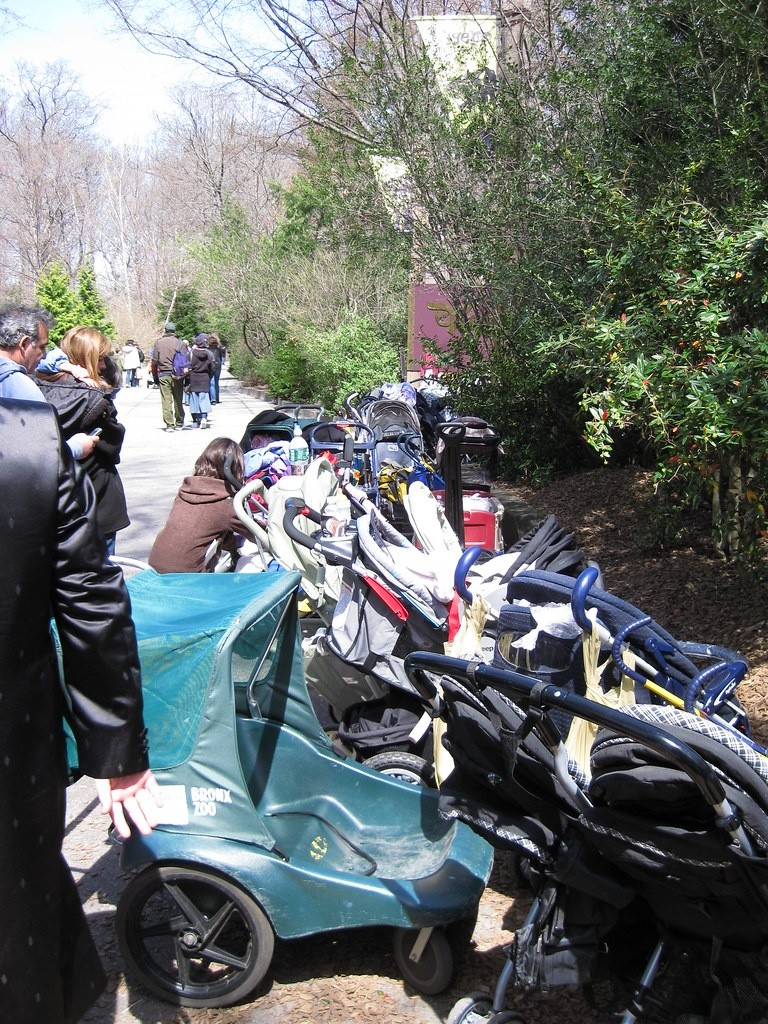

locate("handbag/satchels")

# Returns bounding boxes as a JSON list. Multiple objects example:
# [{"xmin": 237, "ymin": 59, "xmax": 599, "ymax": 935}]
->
[{"xmin": 133, "ymin": 368, "xmax": 143, "ymax": 380}]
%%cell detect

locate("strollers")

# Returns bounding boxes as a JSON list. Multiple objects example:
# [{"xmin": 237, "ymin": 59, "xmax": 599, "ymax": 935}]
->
[
  {"xmin": 52, "ymin": 556, "xmax": 499, "ymax": 1009},
  {"xmin": 398, "ymin": 650, "xmax": 766, "ymax": 1024},
  {"xmin": 206, "ymin": 384, "xmax": 755, "ymax": 736}
]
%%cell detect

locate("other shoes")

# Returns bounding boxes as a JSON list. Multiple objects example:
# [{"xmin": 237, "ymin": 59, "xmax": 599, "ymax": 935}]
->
[
  {"xmin": 200, "ymin": 418, "xmax": 206, "ymax": 429},
  {"xmin": 191, "ymin": 422, "xmax": 197, "ymax": 428},
  {"xmin": 126, "ymin": 384, "xmax": 131, "ymax": 388},
  {"xmin": 167, "ymin": 428, "xmax": 174, "ymax": 431},
  {"xmin": 212, "ymin": 402, "xmax": 216, "ymax": 405},
  {"xmin": 175, "ymin": 426, "xmax": 183, "ymax": 431}
]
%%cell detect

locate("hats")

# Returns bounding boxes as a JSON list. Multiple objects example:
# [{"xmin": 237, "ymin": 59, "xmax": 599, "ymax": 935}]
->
[
  {"xmin": 165, "ymin": 322, "xmax": 175, "ymax": 333},
  {"xmin": 195, "ymin": 333, "xmax": 210, "ymax": 348}
]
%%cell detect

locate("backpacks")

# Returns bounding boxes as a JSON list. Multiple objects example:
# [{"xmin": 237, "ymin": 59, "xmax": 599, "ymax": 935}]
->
[{"xmin": 171, "ymin": 340, "xmax": 191, "ymax": 380}]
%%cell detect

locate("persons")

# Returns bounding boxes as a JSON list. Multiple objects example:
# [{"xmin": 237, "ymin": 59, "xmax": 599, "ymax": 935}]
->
[
  {"xmin": 0, "ymin": 305, "xmax": 166, "ymax": 1024},
  {"xmin": 147, "ymin": 321, "xmax": 226, "ymax": 432},
  {"xmin": 148, "ymin": 437, "xmax": 267, "ymax": 574}
]
